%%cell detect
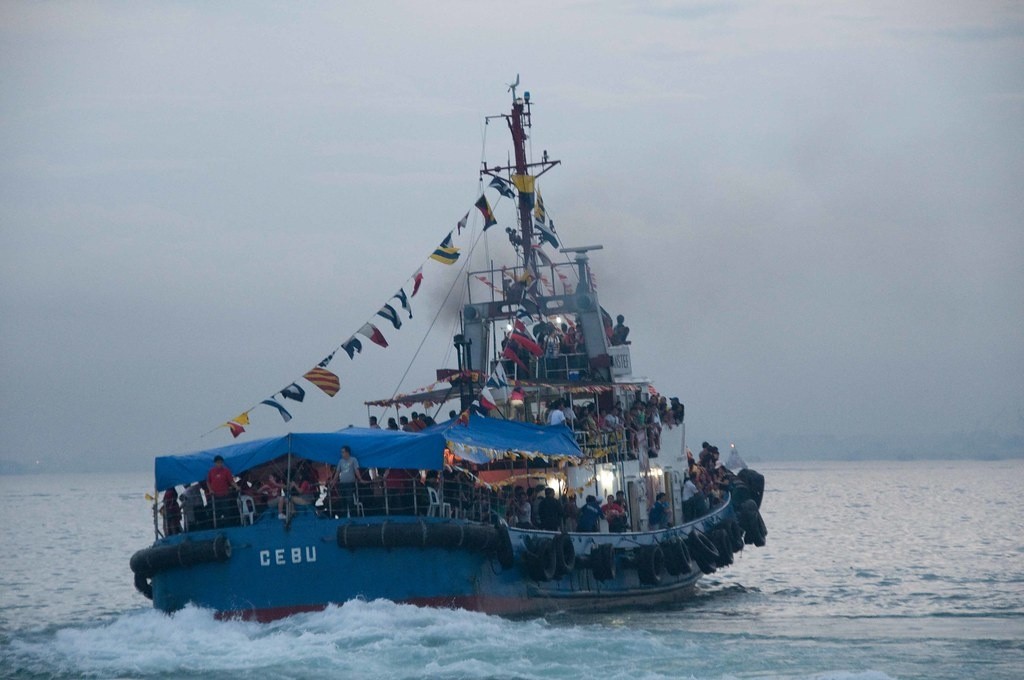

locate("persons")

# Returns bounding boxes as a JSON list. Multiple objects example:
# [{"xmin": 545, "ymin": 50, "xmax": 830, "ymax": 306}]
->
[
  {"xmin": 163, "ymin": 480, "xmax": 207, "ymax": 536},
  {"xmin": 551, "ymin": 395, "xmax": 685, "ymax": 459},
  {"xmin": 682, "ymin": 442, "xmax": 729, "ymax": 523},
  {"xmin": 259, "ymin": 468, "xmax": 319, "ymax": 519},
  {"xmin": 540, "ymin": 314, "xmax": 636, "ymax": 357},
  {"xmin": 331, "ymin": 446, "xmax": 371, "ymax": 519},
  {"xmin": 460, "ymin": 484, "xmax": 630, "ymax": 534},
  {"xmin": 649, "ymin": 492, "xmax": 671, "ymax": 531},
  {"xmin": 208, "ymin": 455, "xmax": 242, "ymax": 528}
]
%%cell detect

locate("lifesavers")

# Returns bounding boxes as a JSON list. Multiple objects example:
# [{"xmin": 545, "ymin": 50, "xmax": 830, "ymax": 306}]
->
[
  {"xmin": 490, "ymin": 519, "xmax": 745, "ymax": 586},
  {"xmin": 739, "ymin": 468, "xmax": 769, "ymax": 547}
]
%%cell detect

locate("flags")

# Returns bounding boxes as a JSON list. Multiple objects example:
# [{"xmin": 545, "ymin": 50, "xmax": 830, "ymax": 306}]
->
[{"xmin": 224, "ymin": 175, "xmax": 557, "ymax": 438}]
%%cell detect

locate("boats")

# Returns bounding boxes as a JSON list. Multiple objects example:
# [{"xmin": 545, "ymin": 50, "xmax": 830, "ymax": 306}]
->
[
  {"xmin": 129, "ymin": 73, "xmax": 768, "ymax": 623},
  {"xmin": 725, "ymin": 446, "xmax": 749, "ymax": 470}
]
